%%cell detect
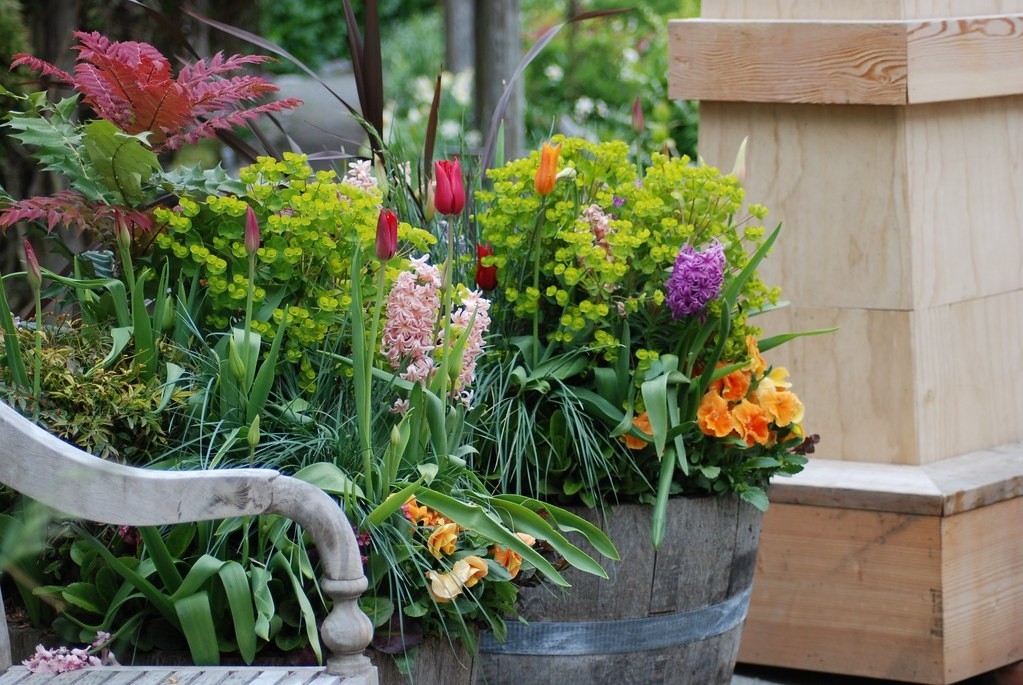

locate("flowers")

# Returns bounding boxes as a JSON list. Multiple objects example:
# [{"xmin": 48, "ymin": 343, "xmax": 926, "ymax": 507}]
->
[{"xmin": 0, "ymin": 0, "xmax": 838, "ymax": 674}]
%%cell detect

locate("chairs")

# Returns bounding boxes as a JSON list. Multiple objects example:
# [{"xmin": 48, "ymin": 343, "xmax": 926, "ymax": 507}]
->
[{"xmin": 0, "ymin": 401, "xmax": 381, "ymax": 685}]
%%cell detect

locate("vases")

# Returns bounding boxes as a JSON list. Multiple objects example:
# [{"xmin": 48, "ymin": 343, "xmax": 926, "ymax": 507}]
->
[{"xmin": 372, "ymin": 496, "xmax": 762, "ymax": 684}]
